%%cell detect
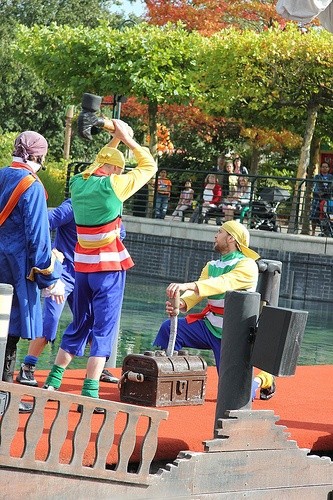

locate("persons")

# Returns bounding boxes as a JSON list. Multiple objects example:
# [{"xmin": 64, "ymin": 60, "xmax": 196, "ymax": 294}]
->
[
  {"xmin": 16, "ymin": 119, "xmax": 333, "ymax": 416},
  {"xmin": 0, "ymin": 131, "xmax": 66, "ymax": 418}
]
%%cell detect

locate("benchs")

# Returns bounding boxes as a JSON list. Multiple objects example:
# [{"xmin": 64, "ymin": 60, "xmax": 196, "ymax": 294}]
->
[{"xmin": 182, "ymin": 207, "xmax": 247, "ymax": 226}]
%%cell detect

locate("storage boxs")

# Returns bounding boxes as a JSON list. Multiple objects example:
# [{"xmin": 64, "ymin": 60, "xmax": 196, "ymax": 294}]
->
[{"xmin": 120, "ymin": 354, "xmax": 207, "ymax": 407}]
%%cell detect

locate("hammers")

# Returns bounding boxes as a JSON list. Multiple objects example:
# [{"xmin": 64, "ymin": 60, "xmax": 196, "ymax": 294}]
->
[{"xmin": 78, "ymin": 93, "xmax": 133, "ymax": 141}]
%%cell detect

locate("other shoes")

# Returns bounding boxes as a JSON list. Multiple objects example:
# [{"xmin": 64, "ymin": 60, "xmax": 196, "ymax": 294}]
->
[
  {"xmin": 0, "ymin": 397, "xmax": 35, "ymax": 414},
  {"xmin": 40, "ymin": 384, "xmax": 59, "ymax": 402},
  {"xmin": 258, "ymin": 374, "xmax": 276, "ymax": 401},
  {"xmin": 101, "ymin": 370, "xmax": 119, "ymax": 384},
  {"xmin": 77, "ymin": 405, "xmax": 105, "ymax": 415},
  {"xmin": 16, "ymin": 363, "xmax": 39, "ymax": 386}
]
